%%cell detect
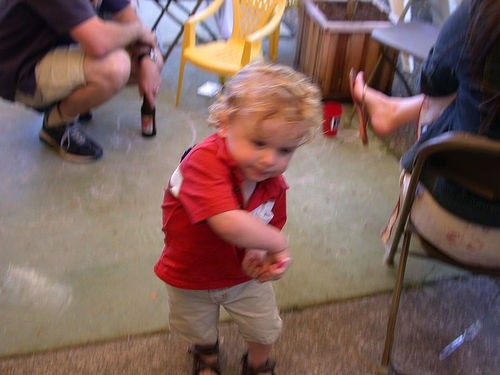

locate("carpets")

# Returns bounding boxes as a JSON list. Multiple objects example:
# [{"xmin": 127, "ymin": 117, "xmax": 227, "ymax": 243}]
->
[{"xmin": 0, "ymin": 274, "xmax": 500, "ymax": 375}]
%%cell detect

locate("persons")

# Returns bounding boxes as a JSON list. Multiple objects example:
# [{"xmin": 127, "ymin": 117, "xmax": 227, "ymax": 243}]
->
[
  {"xmin": 0, "ymin": 0, "xmax": 163, "ymax": 162},
  {"xmin": 352, "ymin": 0, "xmax": 500, "ymax": 268},
  {"xmin": 153, "ymin": 61, "xmax": 324, "ymax": 375}
]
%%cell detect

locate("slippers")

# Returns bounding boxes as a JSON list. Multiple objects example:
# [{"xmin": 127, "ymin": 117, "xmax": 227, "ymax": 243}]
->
[{"xmin": 350, "ymin": 67, "xmax": 369, "ymax": 147}]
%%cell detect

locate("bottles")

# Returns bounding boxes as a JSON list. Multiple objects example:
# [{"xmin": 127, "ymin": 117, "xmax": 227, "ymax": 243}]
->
[{"xmin": 141, "ymin": 93, "xmax": 156, "ymax": 137}]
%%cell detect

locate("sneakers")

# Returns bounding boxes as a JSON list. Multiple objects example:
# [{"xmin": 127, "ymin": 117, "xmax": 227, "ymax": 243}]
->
[{"xmin": 39, "ymin": 114, "xmax": 103, "ymax": 160}]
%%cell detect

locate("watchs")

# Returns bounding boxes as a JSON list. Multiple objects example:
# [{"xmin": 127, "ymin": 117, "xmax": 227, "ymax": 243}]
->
[{"xmin": 135, "ymin": 49, "xmax": 158, "ymax": 64}]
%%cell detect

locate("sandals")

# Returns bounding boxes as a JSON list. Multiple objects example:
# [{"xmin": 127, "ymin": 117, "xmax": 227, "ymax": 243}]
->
[
  {"xmin": 243, "ymin": 351, "xmax": 276, "ymax": 375},
  {"xmin": 192, "ymin": 341, "xmax": 220, "ymax": 375}
]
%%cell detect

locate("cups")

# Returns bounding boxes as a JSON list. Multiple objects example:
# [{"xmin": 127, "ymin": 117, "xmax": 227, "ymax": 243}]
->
[{"xmin": 322, "ymin": 98, "xmax": 342, "ymax": 136}]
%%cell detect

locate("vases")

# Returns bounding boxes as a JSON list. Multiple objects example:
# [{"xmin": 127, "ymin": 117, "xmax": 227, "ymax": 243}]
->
[{"xmin": 295, "ymin": 0, "xmax": 399, "ymax": 101}]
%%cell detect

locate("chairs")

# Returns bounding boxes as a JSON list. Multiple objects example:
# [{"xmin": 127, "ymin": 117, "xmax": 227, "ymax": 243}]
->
[
  {"xmin": 150, "ymin": 0, "xmax": 290, "ymax": 107},
  {"xmin": 381, "ymin": 134, "xmax": 500, "ymax": 375},
  {"xmin": 347, "ymin": 0, "xmax": 460, "ymax": 125}
]
